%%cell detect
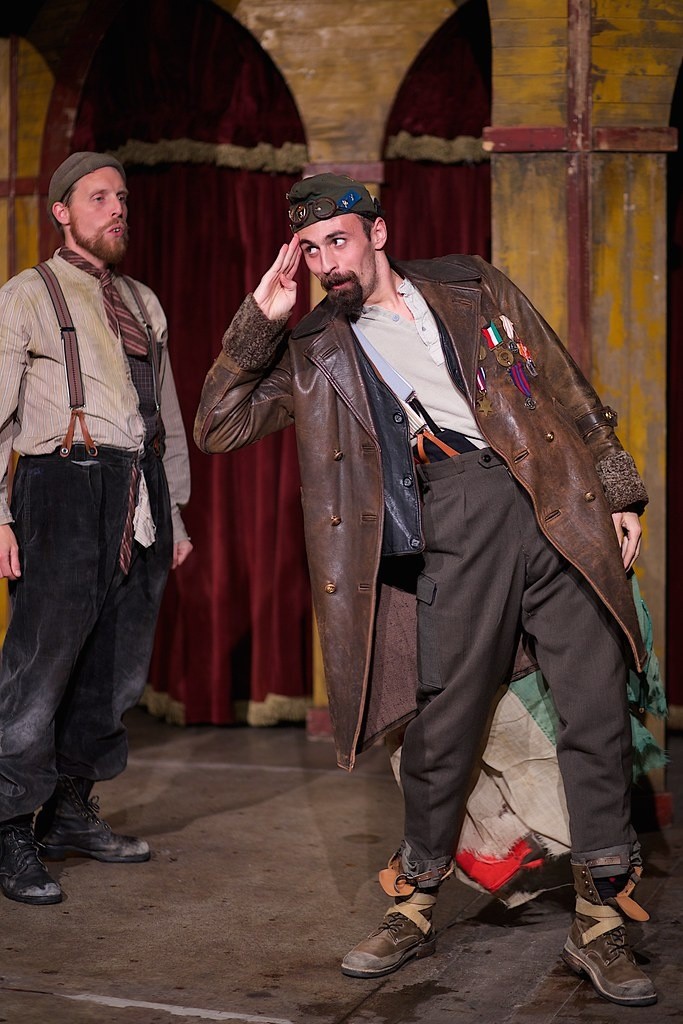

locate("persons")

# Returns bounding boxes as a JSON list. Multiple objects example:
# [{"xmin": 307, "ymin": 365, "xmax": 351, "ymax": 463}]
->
[
  {"xmin": 0, "ymin": 148, "xmax": 196, "ymax": 908},
  {"xmin": 188, "ymin": 170, "xmax": 663, "ymax": 1006}
]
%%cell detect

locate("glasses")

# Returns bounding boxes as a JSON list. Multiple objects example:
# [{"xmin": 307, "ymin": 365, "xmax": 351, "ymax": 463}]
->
[{"xmin": 288, "ymin": 196, "xmax": 375, "ymax": 228}]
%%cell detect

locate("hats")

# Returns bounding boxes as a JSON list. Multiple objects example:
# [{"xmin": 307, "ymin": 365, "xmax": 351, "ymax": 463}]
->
[
  {"xmin": 47, "ymin": 151, "xmax": 125, "ymax": 227},
  {"xmin": 288, "ymin": 172, "xmax": 378, "ymax": 234}
]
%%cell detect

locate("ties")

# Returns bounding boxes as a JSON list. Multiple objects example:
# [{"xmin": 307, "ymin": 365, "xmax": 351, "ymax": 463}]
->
[{"xmin": 58, "ymin": 246, "xmax": 149, "ymax": 356}]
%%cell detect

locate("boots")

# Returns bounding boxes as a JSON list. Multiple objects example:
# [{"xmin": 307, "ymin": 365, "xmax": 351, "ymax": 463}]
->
[
  {"xmin": 562, "ymin": 859, "xmax": 657, "ymax": 1006},
  {"xmin": 341, "ymin": 848, "xmax": 460, "ymax": 976},
  {"xmin": 35, "ymin": 775, "xmax": 151, "ymax": 863},
  {"xmin": 0, "ymin": 813, "xmax": 63, "ymax": 906}
]
